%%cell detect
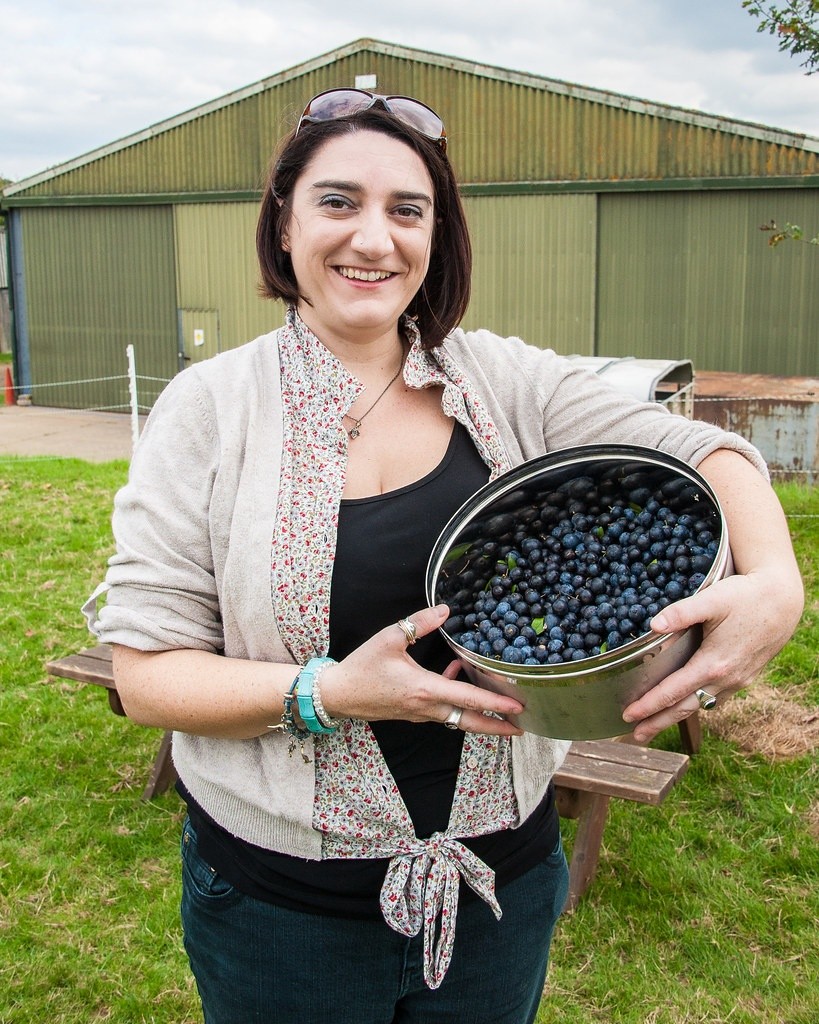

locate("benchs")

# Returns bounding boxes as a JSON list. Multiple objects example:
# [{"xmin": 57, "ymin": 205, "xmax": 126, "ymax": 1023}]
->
[{"xmin": 45, "ymin": 643, "xmax": 688, "ymax": 914}]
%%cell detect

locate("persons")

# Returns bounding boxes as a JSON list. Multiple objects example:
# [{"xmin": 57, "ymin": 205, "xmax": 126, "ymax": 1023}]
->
[{"xmin": 80, "ymin": 100, "xmax": 805, "ymax": 1024}]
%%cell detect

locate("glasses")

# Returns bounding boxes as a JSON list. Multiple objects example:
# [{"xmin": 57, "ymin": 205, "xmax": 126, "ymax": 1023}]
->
[{"xmin": 294, "ymin": 87, "xmax": 448, "ymax": 158}]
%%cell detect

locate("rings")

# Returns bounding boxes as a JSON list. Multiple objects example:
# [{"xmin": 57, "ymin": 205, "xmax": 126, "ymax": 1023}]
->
[
  {"xmin": 397, "ymin": 616, "xmax": 421, "ymax": 645},
  {"xmin": 695, "ymin": 688, "xmax": 717, "ymax": 710},
  {"xmin": 444, "ymin": 707, "xmax": 463, "ymax": 730}
]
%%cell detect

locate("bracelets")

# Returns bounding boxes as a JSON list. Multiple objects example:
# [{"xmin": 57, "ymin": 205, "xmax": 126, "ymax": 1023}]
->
[{"xmin": 266, "ymin": 657, "xmax": 343, "ymax": 766}]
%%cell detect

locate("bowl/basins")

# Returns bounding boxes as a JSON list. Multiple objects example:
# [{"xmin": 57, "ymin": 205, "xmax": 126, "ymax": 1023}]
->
[{"xmin": 424, "ymin": 442, "xmax": 736, "ymax": 744}]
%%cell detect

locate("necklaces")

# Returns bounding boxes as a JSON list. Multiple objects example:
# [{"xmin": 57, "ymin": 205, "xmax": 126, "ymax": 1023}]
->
[{"xmin": 346, "ymin": 339, "xmax": 405, "ymax": 439}]
